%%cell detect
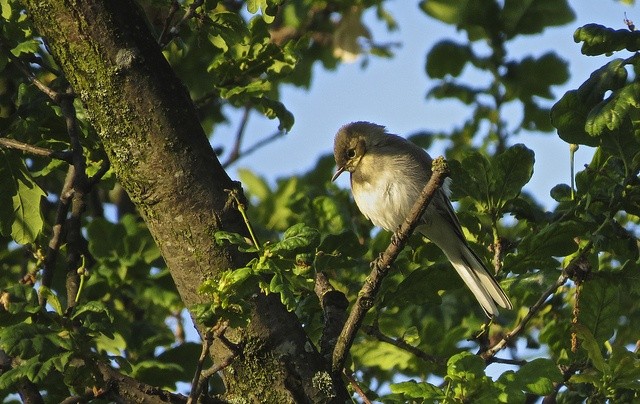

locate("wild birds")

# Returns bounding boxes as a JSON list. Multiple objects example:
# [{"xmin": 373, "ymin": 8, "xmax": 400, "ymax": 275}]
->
[{"xmin": 330, "ymin": 120, "xmax": 513, "ymax": 319}]
